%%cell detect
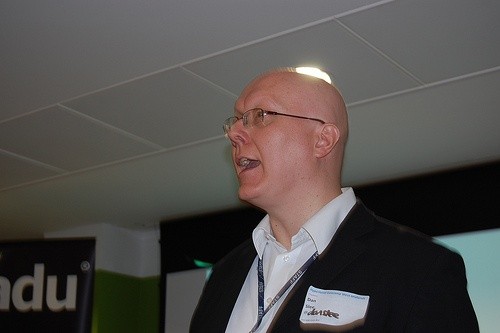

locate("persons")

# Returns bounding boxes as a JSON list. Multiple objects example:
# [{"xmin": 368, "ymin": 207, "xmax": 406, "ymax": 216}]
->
[{"xmin": 189, "ymin": 71, "xmax": 481, "ymax": 333}]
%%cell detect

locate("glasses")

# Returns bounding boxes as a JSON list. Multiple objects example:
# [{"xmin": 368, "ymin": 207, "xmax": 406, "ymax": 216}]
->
[{"xmin": 221, "ymin": 108, "xmax": 329, "ymax": 135}]
